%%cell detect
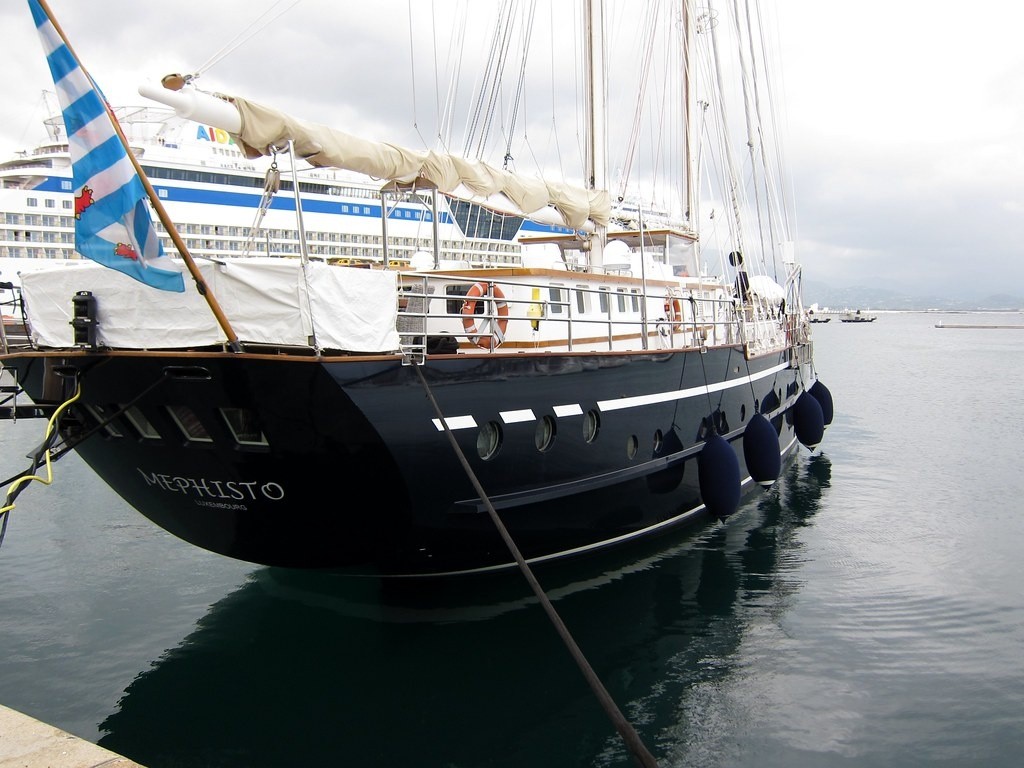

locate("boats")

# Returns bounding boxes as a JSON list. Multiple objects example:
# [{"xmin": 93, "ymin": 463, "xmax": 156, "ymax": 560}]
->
[
  {"xmin": 0, "ymin": 94, "xmax": 685, "ymax": 351},
  {"xmin": 805, "ymin": 308, "xmax": 831, "ymax": 324},
  {"xmin": 840, "ymin": 308, "xmax": 878, "ymax": 323}
]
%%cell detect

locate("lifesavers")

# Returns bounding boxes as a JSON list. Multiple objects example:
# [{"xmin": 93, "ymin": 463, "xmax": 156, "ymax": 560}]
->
[
  {"xmin": 460, "ymin": 284, "xmax": 507, "ymax": 347},
  {"xmin": 666, "ymin": 298, "xmax": 682, "ymax": 331}
]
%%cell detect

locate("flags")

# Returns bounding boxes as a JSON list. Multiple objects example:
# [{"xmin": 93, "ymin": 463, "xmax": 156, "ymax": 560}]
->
[{"xmin": 29, "ymin": 1, "xmax": 186, "ymax": 292}]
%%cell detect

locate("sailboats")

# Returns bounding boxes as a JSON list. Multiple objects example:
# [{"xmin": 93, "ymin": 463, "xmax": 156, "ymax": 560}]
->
[{"xmin": 0, "ymin": 1, "xmax": 837, "ymax": 636}]
